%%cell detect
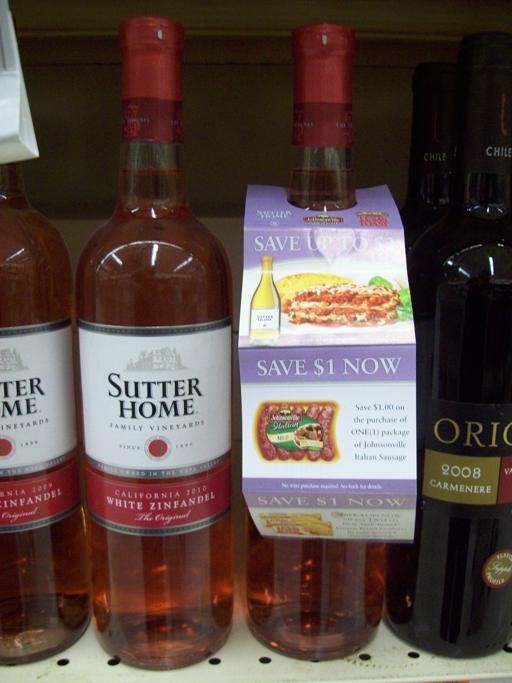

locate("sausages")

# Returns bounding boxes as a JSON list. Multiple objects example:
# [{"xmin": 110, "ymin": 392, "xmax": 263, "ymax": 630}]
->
[{"xmin": 257, "ymin": 403, "xmax": 335, "ymax": 462}]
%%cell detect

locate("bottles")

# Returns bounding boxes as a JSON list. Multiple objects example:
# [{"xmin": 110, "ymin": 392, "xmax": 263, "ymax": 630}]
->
[
  {"xmin": 398, "ymin": 62, "xmax": 468, "ymax": 253},
  {"xmin": 2, "ymin": 164, "xmax": 94, "ymax": 666},
  {"xmin": 243, "ymin": 20, "xmax": 390, "ymax": 662},
  {"xmin": 77, "ymin": 15, "xmax": 243, "ymax": 671},
  {"xmin": 385, "ymin": 30, "xmax": 511, "ymax": 662}
]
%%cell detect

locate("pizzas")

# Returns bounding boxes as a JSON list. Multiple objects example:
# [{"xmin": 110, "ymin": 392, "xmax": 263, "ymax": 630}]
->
[{"xmin": 280, "ymin": 283, "xmax": 401, "ymax": 329}]
